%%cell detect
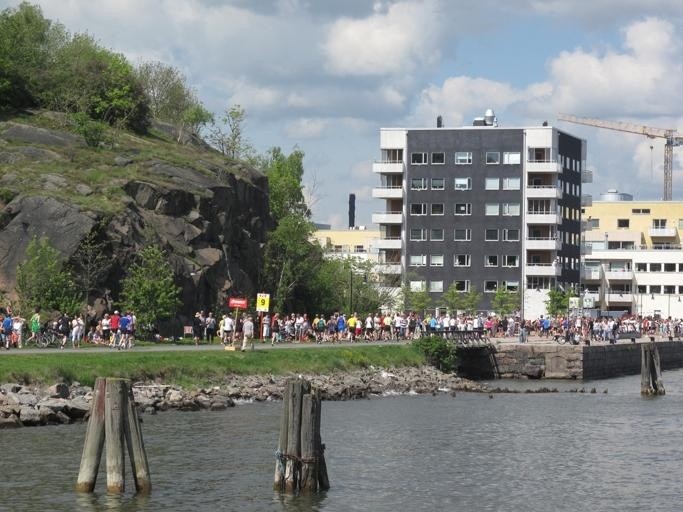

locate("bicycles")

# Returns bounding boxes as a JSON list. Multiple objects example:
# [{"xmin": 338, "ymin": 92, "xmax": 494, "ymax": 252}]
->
[{"xmin": 36, "ymin": 328, "xmax": 61, "ymax": 348}]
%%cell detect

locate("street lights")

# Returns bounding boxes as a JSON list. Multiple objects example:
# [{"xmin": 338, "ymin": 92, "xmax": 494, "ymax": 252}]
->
[
  {"xmin": 552, "ymin": 259, "xmax": 565, "ymax": 293},
  {"xmin": 669, "ymin": 294, "xmax": 680, "ymax": 316},
  {"xmin": 640, "ymin": 292, "xmax": 654, "ymax": 333},
  {"xmin": 523, "ymin": 281, "xmax": 540, "ymax": 320},
  {"xmin": 350, "ymin": 271, "xmax": 367, "ymax": 314},
  {"xmin": 174, "ymin": 261, "xmax": 196, "ymax": 341}
]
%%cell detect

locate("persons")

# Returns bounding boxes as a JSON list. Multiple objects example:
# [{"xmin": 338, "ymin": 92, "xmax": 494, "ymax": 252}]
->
[
  {"xmin": 1, "ymin": 303, "xmax": 138, "ymax": 351},
  {"xmin": 143, "ymin": 308, "xmax": 682, "ymax": 350}
]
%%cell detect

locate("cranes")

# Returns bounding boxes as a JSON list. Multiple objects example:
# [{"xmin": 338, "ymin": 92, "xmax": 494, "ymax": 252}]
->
[{"xmin": 558, "ymin": 114, "xmax": 683, "ymax": 201}]
